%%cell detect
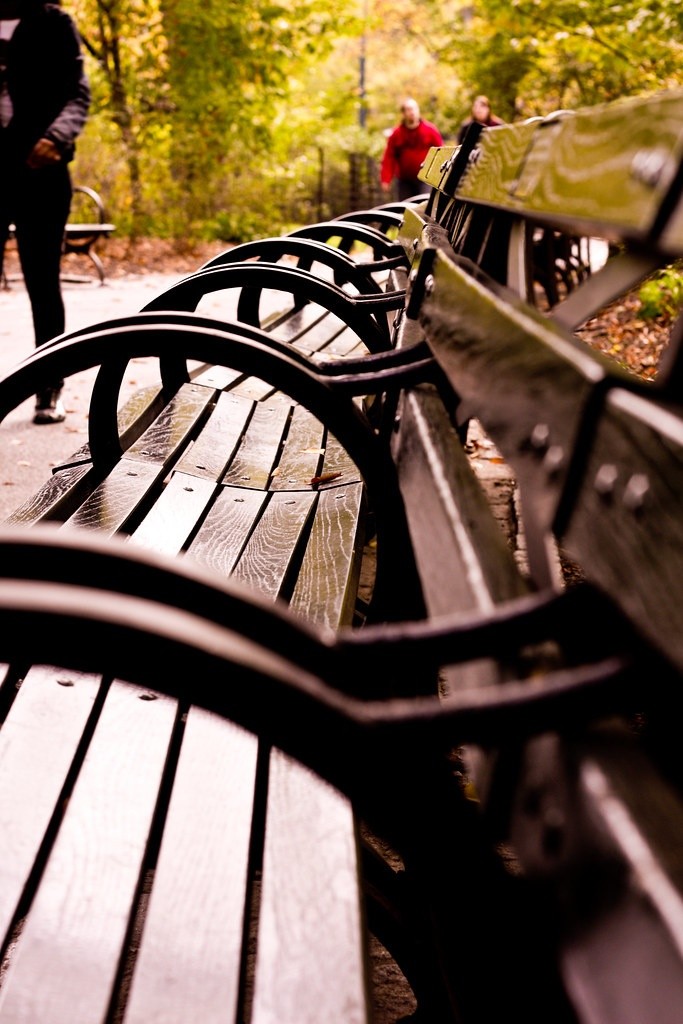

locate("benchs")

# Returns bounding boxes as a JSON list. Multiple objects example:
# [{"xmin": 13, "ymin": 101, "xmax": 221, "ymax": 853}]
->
[{"xmin": 1, "ymin": 89, "xmax": 683, "ymax": 1023}]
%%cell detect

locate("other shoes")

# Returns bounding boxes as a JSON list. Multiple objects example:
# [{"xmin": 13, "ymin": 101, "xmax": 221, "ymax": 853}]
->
[{"xmin": 33, "ymin": 398, "xmax": 65, "ymax": 423}]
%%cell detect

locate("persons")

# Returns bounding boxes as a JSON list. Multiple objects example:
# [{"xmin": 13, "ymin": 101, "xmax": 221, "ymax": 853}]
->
[
  {"xmin": 458, "ymin": 95, "xmax": 507, "ymax": 140},
  {"xmin": 381, "ymin": 99, "xmax": 447, "ymax": 201},
  {"xmin": 0, "ymin": 0, "xmax": 92, "ymax": 424}
]
